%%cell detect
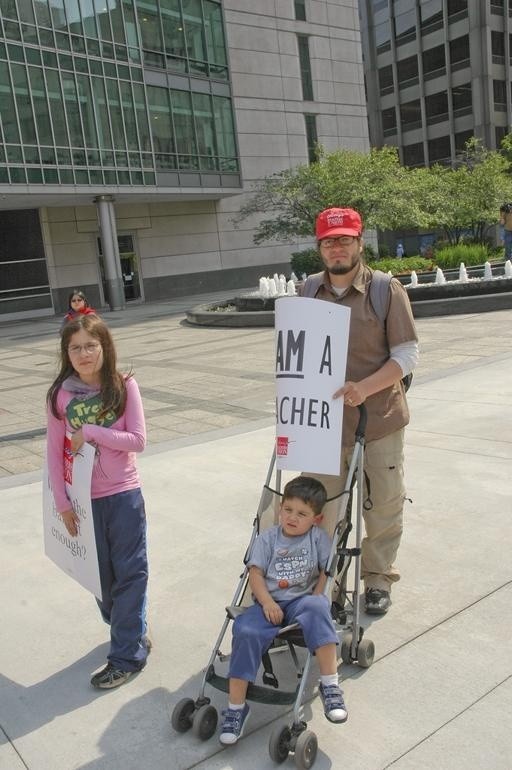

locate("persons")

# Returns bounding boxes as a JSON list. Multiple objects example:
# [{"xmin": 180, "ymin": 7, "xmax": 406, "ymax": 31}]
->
[
  {"xmin": 59, "ymin": 289, "xmax": 97, "ymax": 335},
  {"xmin": 297, "ymin": 208, "xmax": 420, "ymax": 615},
  {"xmin": 45, "ymin": 314, "xmax": 149, "ymax": 689},
  {"xmin": 396, "ymin": 243, "xmax": 404, "ymax": 258},
  {"xmin": 500, "ymin": 205, "xmax": 512, "ymax": 263},
  {"xmin": 218, "ymin": 476, "xmax": 349, "ymax": 746}
]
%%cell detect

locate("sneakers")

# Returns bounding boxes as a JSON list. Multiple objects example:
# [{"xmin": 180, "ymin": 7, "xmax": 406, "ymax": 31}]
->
[
  {"xmin": 219, "ymin": 701, "xmax": 252, "ymax": 747},
  {"xmin": 363, "ymin": 588, "xmax": 391, "ymax": 615},
  {"xmin": 317, "ymin": 681, "xmax": 348, "ymax": 724},
  {"xmin": 90, "ymin": 661, "xmax": 137, "ymax": 689}
]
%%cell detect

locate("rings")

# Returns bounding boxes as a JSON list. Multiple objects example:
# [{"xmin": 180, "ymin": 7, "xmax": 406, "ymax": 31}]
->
[{"xmin": 348, "ymin": 398, "xmax": 352, "ymax": 404}]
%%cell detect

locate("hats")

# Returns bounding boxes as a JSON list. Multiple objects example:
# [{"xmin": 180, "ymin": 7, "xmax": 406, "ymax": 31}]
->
[{"xmin": 314, "ymin": 208, "xmax": 362, "ymax": 241}]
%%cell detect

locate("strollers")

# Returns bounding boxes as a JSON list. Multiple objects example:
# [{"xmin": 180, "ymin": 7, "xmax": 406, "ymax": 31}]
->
[{"xmin": 166, "ymin": 375, "xmax": 381, "ymax": 769}]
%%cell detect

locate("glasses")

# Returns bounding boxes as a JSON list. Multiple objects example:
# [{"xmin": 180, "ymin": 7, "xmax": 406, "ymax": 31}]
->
[{"xmin": 317, "ymin": 236, "xmax": 356, "ymax": 248}]
